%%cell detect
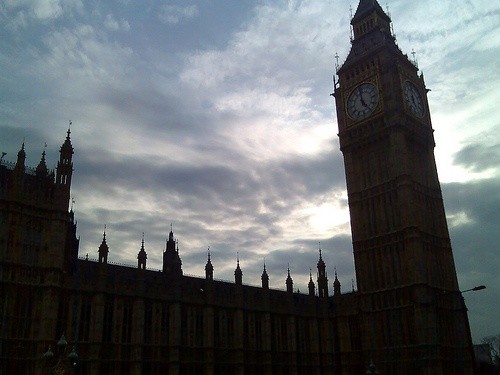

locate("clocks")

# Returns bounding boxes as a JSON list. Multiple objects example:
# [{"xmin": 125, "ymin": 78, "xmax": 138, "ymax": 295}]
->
[
  {"xmin": 403, "ymin": 78, "xmax": 427, "ymax": 119},
  {"xmin": 344, "ymin": 81, "xmax": 380, "ymax": 121}
]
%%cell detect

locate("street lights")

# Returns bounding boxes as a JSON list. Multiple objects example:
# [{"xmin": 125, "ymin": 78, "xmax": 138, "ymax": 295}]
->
[{"xmin": 431, "ymin": 285, "xmax": 488, "ymax": 375}]
